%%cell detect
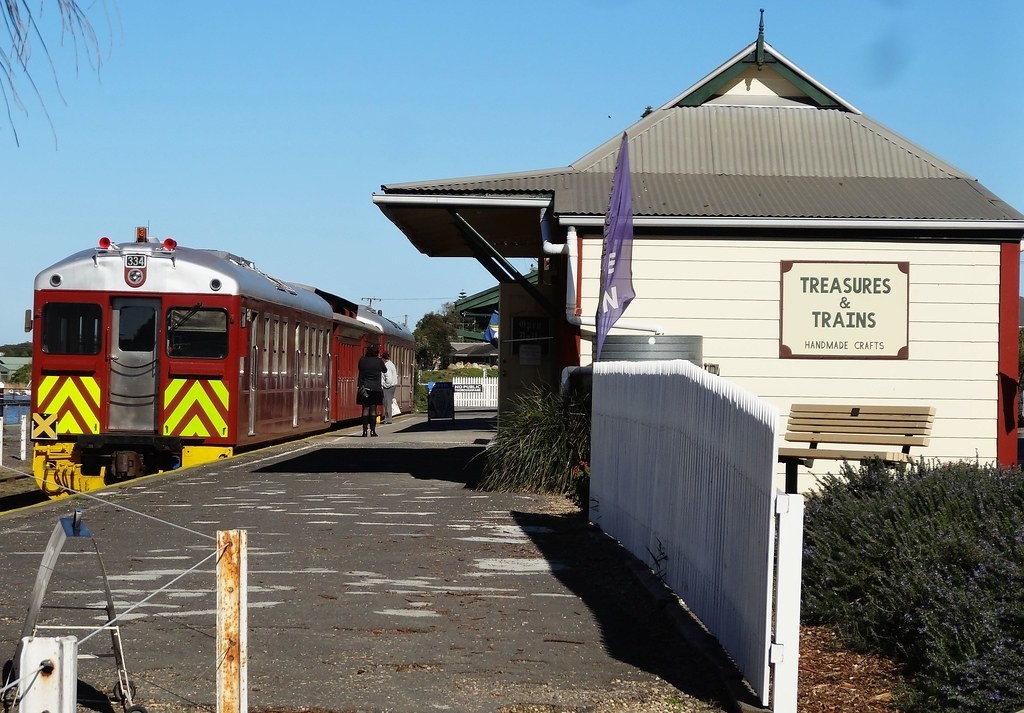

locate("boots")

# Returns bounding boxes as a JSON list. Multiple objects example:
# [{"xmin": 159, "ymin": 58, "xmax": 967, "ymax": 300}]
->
[
  {"xmin": 362, "ymin": 416, "xmax": 368, "ymax": 437},
  {"xmin": 369, "ymin": 415, "xmax": 378, "ymax": 437}
]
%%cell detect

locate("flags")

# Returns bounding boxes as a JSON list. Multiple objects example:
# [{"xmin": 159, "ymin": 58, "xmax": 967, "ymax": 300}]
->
[
  {"xmin": 482, "ymin": 310, "xmax": 499, "ymax": 343},
  {"xmin": 596, "ymin": 129, "xmax": 636, "ymax": 366}
]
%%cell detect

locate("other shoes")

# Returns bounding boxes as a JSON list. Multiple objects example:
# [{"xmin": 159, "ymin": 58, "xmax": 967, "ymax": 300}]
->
[{"xmin": 380, "ymin": 420, "xmax": 392, "ymax": 424}]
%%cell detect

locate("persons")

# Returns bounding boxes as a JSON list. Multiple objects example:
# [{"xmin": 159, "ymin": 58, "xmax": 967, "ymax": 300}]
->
[
  {"xmin": 355, "ymin": 344, "xmax": 387, "ymax": 437},
  {"xmin": 380, "ymin": 352, "xmax": 397, "ymax": 424}
]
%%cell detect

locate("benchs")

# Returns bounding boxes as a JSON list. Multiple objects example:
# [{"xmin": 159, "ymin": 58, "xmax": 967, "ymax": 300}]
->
[{"xmin": 778, "ymin": 404, "xmax": 937, "ymax": 494}]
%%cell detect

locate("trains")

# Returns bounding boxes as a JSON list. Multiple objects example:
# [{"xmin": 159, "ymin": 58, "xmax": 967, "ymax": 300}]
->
[{"xmin": 26, "ymin": 226, "xmax": 416, "ymax": 500}]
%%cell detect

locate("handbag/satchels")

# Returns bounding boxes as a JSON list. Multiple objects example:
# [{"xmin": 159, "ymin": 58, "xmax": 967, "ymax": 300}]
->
[
  {"xmin": 384, "ymin": 398, "xmax": 401, "ymax": 416},
  {"xmin": 356, "ymin": 385, "xmax": 371, "ymax": 404}
]
